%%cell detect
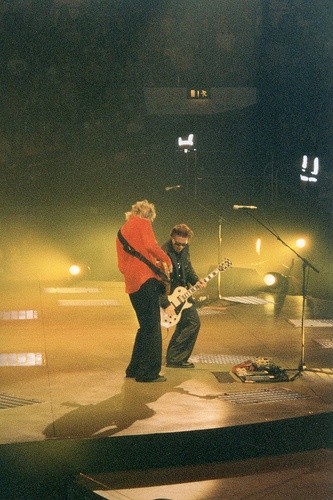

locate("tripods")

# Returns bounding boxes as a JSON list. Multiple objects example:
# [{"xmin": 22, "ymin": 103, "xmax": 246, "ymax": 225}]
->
[{"xmin": 243, "ymin": 210, "xmax": 333, "ymax": 380}]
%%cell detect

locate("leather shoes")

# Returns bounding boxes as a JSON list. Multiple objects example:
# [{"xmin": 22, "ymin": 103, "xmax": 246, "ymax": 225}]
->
[
  {"xmin": 166, "ymin": 360, "xmax": 195, "ymax": 368},
  {"xmin": 125, "ymin": 370, "xmax": 166, "ymax": 382}
]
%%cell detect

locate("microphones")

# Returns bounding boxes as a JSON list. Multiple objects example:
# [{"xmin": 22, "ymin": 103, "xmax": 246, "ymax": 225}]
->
[
  {"xmin": 166, "ymin": 185, "xmax": 181, "ymax": 191},
  {"xmin": 233, "ymin": 205, "xmax": 258, "ymax": 210}
]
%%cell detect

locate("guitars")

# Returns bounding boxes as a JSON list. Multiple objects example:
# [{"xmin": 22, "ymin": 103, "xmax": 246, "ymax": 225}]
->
[
  {"xmin": 159, "ymin": 256, "xmax": 233, "ymax": 330},
  {"xmin": 156, "ymin": 259, "xmax": 171, "ymax": 296}
]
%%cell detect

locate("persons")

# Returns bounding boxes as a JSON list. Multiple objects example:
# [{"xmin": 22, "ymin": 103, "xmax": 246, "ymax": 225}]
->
[
  {"xmin": 158, "ymin": 224, "xmax": 207, "ymax": 368},
  {"xmin": 115, "ymin": 200, "xmax": 174, "ymax": 382}
]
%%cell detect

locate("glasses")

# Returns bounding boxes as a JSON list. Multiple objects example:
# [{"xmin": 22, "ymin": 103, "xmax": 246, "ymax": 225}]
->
[
  {"xmin": 176, "ymin": 262, "xmax": 182, "ymax": 269},
  {"xmin": 174, "ymin": 238, "xmax": 189, "ymax": 248}
]
